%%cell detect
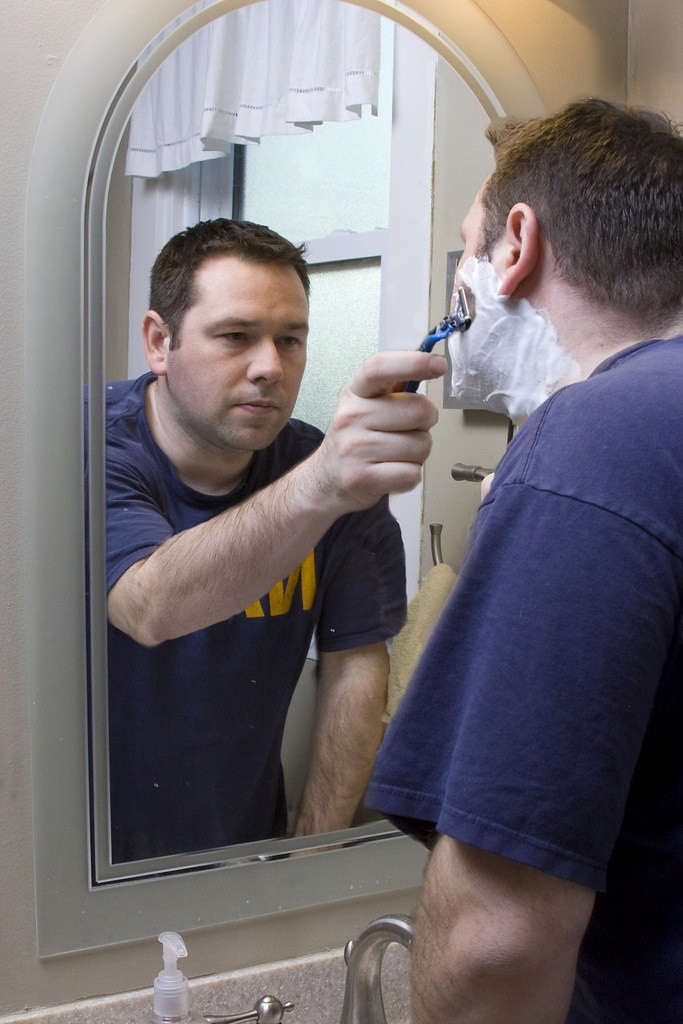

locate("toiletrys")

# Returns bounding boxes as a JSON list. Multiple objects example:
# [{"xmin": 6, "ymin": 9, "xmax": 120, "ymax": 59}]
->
[{"xmin": 151, "ymin": 926, "xmax": 191, "ymax": 1023}]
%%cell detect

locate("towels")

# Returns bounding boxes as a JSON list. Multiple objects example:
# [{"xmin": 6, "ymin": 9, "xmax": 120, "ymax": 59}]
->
[{"xmin": 381, "ymin": 561, "xmax": 459, "ymax": 729}]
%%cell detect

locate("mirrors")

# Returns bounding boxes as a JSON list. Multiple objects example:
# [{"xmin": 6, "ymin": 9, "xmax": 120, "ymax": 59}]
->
[{"xmin": 29, "ymin": 0, "xmax": 552, "ymax": 962}]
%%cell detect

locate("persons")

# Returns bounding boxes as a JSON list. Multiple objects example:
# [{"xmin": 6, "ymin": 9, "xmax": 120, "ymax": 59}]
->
[
  {"xmin": 365, "ymin": 101, "xmax": 683, "ymax": 1024},
  {"xmin": 84, "ymin": 219, "xmax": 448, "ymax": 865}
]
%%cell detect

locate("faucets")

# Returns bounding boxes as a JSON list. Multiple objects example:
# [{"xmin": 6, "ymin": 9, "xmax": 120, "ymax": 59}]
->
[{"xmin": 338, "ymin": 916, "xmax": 411, "ymax": 1023}]
